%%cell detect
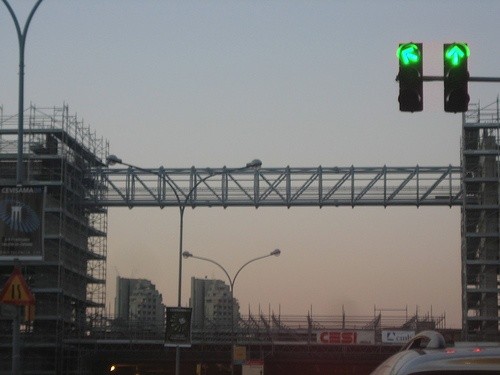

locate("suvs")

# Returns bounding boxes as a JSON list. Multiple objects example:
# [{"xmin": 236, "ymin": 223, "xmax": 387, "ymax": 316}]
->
[{"xmin": 367, "ymin": 329, "xmax": 500, "ymax": 375}]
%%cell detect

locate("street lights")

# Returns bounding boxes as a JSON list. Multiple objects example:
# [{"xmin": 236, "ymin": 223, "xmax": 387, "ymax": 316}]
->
[
  {"xmin": 106, "ymin": 154, "xmax": 263, "ymax": 375},
  {"xmin": 183, "ymin": 247, "xmax": 281, "ymax": 375}
]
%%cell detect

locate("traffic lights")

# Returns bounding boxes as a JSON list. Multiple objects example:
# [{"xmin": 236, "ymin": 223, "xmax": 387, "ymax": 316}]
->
[
  {"xmin": 443, "ymin": 42, "xmax": 470, "ymax": 112},
  {"xmin": 398, "ymin": 41, "xmax": 424, "ymax": 112}
]
